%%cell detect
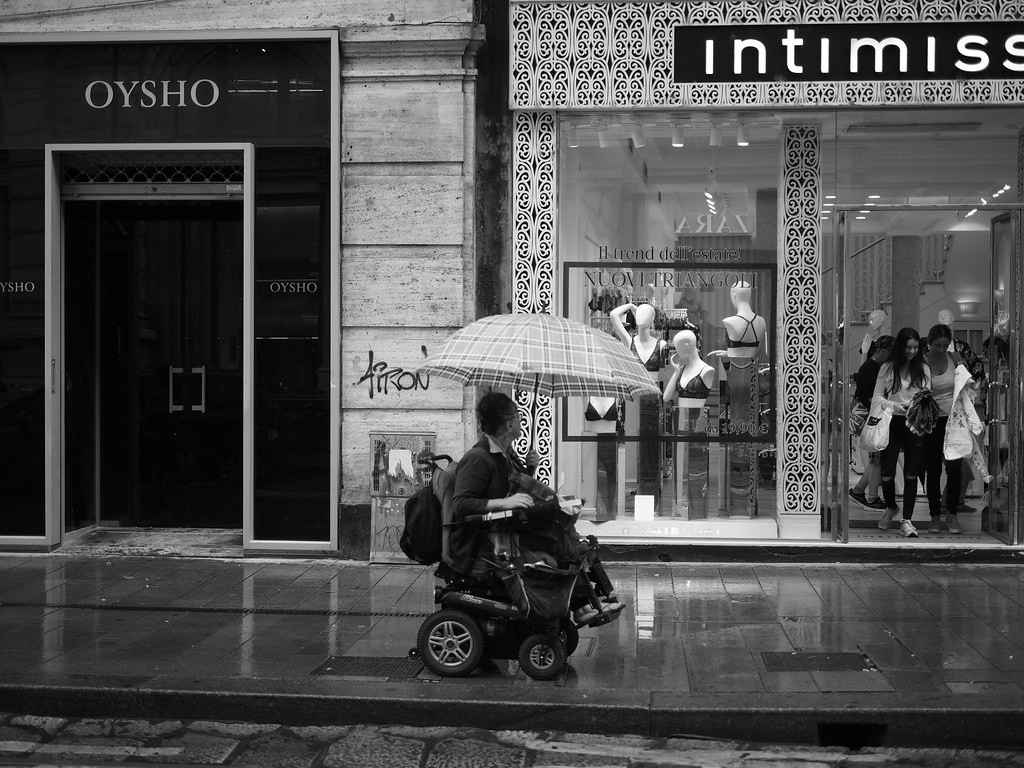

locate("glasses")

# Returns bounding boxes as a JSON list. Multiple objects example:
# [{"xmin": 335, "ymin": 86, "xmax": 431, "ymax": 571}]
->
[{"xmin": 983, "ymin": 350, "xmax": 988, "ymax": 356}]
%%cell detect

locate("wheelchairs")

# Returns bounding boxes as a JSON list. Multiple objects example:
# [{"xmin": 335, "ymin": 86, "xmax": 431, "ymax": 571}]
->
[{"xmin": 409, "ymin": 454, "xmax": 622, "ymax": 682}]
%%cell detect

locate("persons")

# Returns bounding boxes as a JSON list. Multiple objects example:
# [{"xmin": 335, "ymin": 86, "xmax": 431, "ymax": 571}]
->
[
  {"xmin": 937, "ymin": 308, "xmax": 955, "ymax": 338},
  {"xmin": 845, "ymin": 323, "xmax": 1009, "ymax": 537},
  {"xmin": 446, "ymin": 390, "xmax": 627, "ymax": 629},
  {"xmin": 858, "ymin": 309, "xmax": 889, "ymax": 369},
  {"xmin": 707, "ymin": 281, "xmax": 766, "ymax": 357},
  {"xmin": 583, "ymin": 396, "xmax": 617, "ymax": 432},
  {"xmin": 662, "ymin": 329, "xmax": 716, "ymax": 407},
  {"xmin": 609, "ymin": 303, "xmax": 668, "ymax": 381}
]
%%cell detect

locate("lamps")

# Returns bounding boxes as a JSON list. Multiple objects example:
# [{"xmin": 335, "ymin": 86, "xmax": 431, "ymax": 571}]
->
[
  {"xmin": 704, "ymin": 181, "xmax": 723, "ymax": 215},
  {"xmin": 568, "ymin": 119, "xmax": 752, "ymax": 148},
  {"xmin": 956, "ymin": 299, "xmax": 981, "ymax": 318}
]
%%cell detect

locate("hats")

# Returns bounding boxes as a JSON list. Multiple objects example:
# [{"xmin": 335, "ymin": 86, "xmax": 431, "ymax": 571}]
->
[{"xmin": 874, "ymin": 335, "xmax": 896, "ymax": 351}]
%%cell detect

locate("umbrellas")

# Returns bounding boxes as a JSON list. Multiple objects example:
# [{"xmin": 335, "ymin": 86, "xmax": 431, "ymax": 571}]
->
[{"xmin": 413, "ymin": 301, "xmax": 664, "ymax": 478}]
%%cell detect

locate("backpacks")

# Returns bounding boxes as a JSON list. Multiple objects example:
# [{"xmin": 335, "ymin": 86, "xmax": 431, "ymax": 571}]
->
[{"xmin": 399, "ymin": 486, "xmax": 445, "ymax": 569}]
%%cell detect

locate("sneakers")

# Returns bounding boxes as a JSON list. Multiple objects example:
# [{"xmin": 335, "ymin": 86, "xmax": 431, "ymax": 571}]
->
[
  {"xmin": 945, "ymin": 513, "xmax": 963, "ymax": 534},
  {"xmin": 928, "ymin": 515, "xmax": 943, "ymax": 533},
  {"xmin": 864, "ymin": 497, "xmax": 886, "ymax": 512},
  {"xmin": 878, "ymin": 505, "xmax": 900, "ymax": 531},
  {"xmin": 899, "ymin": 519, "xmax": 918, "ymax": 538},
  {"xmin": 848, "ymin": 488, "xmax": 869, "ymax": 507}
]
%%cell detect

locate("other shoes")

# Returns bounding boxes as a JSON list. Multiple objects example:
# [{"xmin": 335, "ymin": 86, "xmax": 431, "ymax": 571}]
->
[{"xmin": 575, "ymin": 602, "xmax": 626, "ymax": 629}]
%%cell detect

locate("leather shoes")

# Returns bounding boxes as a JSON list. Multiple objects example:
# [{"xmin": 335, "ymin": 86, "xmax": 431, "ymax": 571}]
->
[
  {"xmin": 940, "ymin": 508, "xmax": 947, "ymax": 516},
  {"xmin": 953, "ymin": 504, "xmax": 976, "ymax": 513}
]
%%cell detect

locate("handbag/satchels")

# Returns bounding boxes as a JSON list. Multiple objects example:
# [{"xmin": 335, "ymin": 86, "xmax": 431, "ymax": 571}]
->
[
  {"xmin": 858, "ymin": 398, "xmax": 894, "ymax": 452},
  {"xmin": 849, "ymin": 398, "xmax": 870, "ymax": 436}
]
